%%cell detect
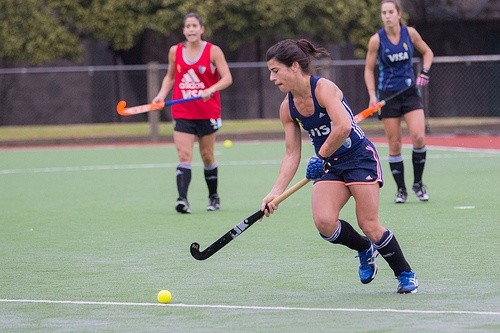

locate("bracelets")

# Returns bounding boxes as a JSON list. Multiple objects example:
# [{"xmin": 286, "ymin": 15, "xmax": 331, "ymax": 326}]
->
[{"xmin": 423, "ymin": 68, "xmax": 430, "ymax": 71}]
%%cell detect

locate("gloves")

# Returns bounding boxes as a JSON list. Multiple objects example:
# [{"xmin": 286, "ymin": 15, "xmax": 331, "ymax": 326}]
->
[
  {"xmin": 415, "ymin": 70, "xmax": 431, "ymax": 89},
  {"xmin": 306, "ymin": 152, "xmax": 329, "ymax": 182}
]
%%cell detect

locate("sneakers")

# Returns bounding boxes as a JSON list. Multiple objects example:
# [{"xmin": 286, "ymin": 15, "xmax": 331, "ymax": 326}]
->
[
  {"xmin": 412, "ymin": 182, "xmax": 429, "ymax": 201},
  {"xmin": 393, "ymin": 270, "xmax": 419, "ymax": 295},
  {"xmin": 355, "ymin": 237, "xmax": 379, "ymax": 284},
  {"xmin": 175, "ymin": 197, "xmax": 192, "ymax": 214},
  {"xmin": 395, "ymin": 187, "xmax": 408, "ymax": 203},
  {"xmin": 206, "ymin": 193, "xmax": 220, "ymax": 211}
]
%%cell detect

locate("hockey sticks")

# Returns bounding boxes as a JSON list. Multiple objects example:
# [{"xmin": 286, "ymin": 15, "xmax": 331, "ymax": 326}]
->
[
  {"xmin": 190, "ymin": 161, "xmax": 332, "ymax": 261},
  {"xmin": 116, "ymin": 93, "xmax": 213, "ymax": 116},
  {"xmin": 355, "ymin": 82, "xmax": 416, "ymax": 124}
]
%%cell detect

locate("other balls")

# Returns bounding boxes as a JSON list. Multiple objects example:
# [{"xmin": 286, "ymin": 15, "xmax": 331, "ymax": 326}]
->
[
  {"xmin": 158, "ymin": 290, "xmax": 172, "ymax": 303},
  {"xmin": 224, "ymin": 140, "xmax": 232, "ymax": 147}
]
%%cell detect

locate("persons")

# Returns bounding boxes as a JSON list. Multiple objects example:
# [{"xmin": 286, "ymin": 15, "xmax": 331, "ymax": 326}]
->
[
  {"xmin": 364, "ymin": 0, "xmax": 434, "ymax": 204},
  {"xmin": 152, "ymin": 13, "xmax": 232, "ymax": 214},
  {"xmin": 258, "ymin": 39, "xmax": 419, "ymax": 294}
]
